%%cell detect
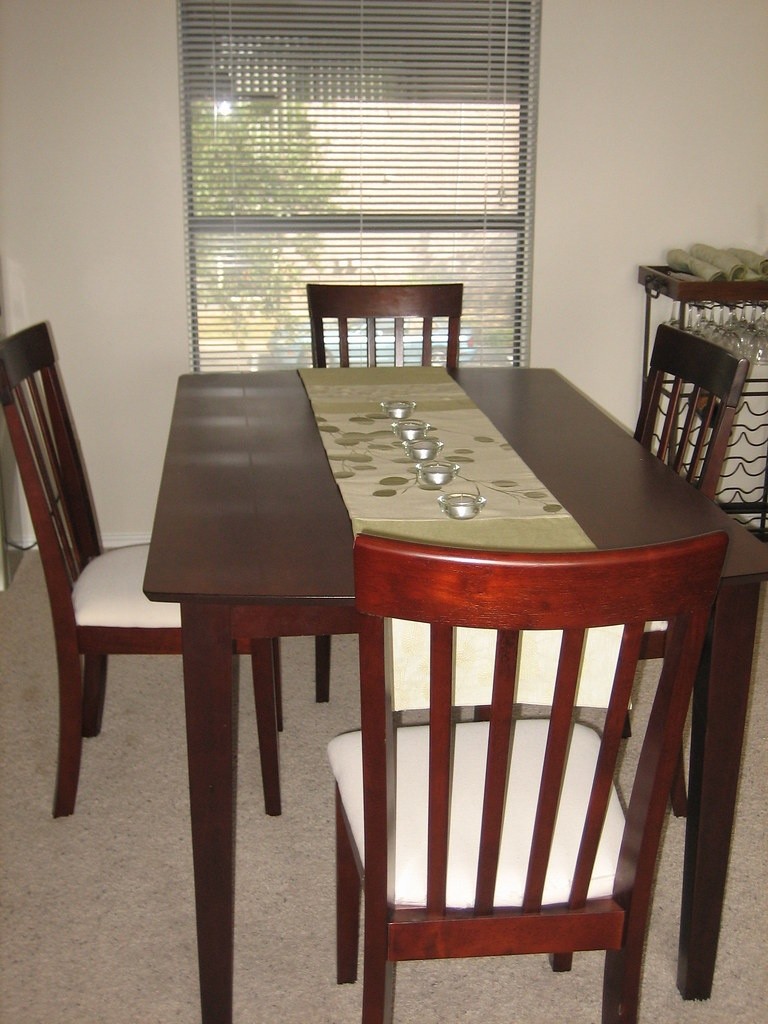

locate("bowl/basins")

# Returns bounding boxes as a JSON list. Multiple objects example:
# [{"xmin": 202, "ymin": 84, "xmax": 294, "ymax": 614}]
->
[
  {"xmin": 415, "ymin": 461, "xmax": 460, "ymax": 485},
  {"xmin": 437, "ymin": 492, "xmax": 485, "ymax": 520},
  {"xmin": 391, "ymin": 419, "xmax": 431, "ymax": 440},
  {"xmin": 402, "ymin": 438, "xmax": 444, "ymax": 461},
  {"xmin": 380, "ymin": 400, "xmax": 416, "ymax": 418}
]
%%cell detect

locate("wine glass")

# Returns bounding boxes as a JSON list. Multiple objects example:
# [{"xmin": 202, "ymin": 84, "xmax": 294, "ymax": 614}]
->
[{"xmin": 664, "ymin": 300, "xmax": 768, "ymax": 366}]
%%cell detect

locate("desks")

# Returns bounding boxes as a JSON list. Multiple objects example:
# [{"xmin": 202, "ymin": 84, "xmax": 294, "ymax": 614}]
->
[{"xmin": 143, "ymin": 366, "xmax": 767, "ymax": 1023}]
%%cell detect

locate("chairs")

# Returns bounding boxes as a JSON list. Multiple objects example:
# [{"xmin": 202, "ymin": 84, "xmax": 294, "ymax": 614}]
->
[
  {"xmin": 326, "ymin": 529, "xmax": 729, "ymax": 1024},
  {"xmin": 0, "ymin": 321, "xmax": 284, "ymax": 819},
  {"xmin": 306, "ymin": 284, "xmax": 465, "ymax": 704},
  {"xmin": 473, "ymin": 323, "xmax": 750, "ymax": 819}
]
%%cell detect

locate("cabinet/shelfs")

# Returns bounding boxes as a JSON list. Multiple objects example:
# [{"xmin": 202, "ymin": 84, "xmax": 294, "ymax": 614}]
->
[{"xmin": 638, "ymin": 263, "xmax": 768, "ymax": 547}]
